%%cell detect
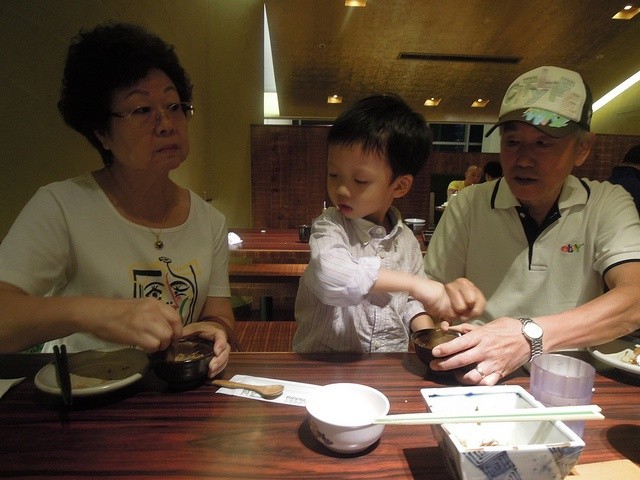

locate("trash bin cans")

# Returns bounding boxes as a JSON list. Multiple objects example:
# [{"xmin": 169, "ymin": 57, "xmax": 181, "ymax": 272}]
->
[{"xmin": 517, "ymin": 316, "xmax": 545, "ymax": 363}]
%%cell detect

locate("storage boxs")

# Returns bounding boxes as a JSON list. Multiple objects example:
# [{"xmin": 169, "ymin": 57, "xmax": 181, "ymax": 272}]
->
[{"xmin": 229, "ymin": 264, "xmax": 311, "ymax": 276}]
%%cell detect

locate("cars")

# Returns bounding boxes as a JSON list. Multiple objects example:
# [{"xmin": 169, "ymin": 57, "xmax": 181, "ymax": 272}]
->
[
  {"xmin": 300, "ymin": 224, "xmax": 312, "ymax": 244},
  {"xmin": 528, "ymin": 352, "xmax": 596, "ymax": 440}
]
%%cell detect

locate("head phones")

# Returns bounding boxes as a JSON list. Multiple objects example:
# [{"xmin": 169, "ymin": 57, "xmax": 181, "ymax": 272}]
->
[
  {"xmin": 53, "ymin": 344, "xmax": 80, "ymax": 430},
  {"xmin": 370, "ymin": 403, "xmax": 606, "ymax": 425}
]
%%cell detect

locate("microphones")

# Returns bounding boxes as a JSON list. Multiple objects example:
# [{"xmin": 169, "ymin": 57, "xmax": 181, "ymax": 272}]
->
[{"xmin": 236, "ymin": 320, "xmax": 301, "ymax": 352}]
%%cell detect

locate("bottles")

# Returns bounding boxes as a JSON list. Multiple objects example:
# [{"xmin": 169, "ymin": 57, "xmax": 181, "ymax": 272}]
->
[{"xmin": 484, "ymin": 65, "xmax": 594, "ymax": 138}]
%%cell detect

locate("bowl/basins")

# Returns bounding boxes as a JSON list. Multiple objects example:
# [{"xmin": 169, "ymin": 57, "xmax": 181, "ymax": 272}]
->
[{"xmin": 108, "ymin": 166, "xmax": 169, "ymax": 249}]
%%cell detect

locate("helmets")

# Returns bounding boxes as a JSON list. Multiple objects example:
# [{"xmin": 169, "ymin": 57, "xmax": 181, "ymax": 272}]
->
[
  {"xmin": 20, "ymin": 347, "xmax": 154, "ymax": 395},
  {"xmin": 419, "ymin": 230, "xmax": 434, "ymax": 249},
  {"xmin": 590, "ymin": 332, "xmax": 639, "ymax": 375}
]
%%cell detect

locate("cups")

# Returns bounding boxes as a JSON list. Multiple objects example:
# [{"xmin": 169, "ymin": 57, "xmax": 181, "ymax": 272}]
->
[{"xmin": 115, "ymin": 102, "xmax": 194, "ymax": 121}]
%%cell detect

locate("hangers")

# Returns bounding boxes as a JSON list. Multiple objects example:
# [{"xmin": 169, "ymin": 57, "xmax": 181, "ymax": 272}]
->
[{"xmin": 212, "ymin": 372, "xmax": 285, "ymax": 399}]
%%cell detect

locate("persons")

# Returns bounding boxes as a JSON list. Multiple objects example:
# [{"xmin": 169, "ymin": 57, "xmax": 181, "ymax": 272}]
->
[
  {"xmin": 447, "ymin": 164, "xmax": 482, "ymax": 201},
  {"xmin": 0, "ymin": 21, "xmax": 235, "ymax": 380},
  {"xmin": 424, "ymin": 66, "xmax": 639, "ymax": 386},
  {"xmin": 293, "ymin": 91, "xmax": 448, "ymax": 355},
  {"xmin": 484, "ymin": 160, "xmax": 503, "ymax": 180}
]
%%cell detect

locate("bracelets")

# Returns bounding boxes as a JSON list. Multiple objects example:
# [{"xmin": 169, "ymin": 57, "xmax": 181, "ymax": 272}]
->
[
  {"xmin": 306, "ymin": 382, "xmax": 391, "ymax": 455},
  {"xmin": 404, "ymin": 216, "xmax": 426, "ymax": 233},
  {"xmin": 405, "ymin": 328, "xmax": 464, "ymax": 378},
  {"xmin": 420, "ymin": 383, "xmax": 587, "ymax": 480},
  {"xmin": 150, "ymin": 336, "xmax": 221, "ymax": 390}
]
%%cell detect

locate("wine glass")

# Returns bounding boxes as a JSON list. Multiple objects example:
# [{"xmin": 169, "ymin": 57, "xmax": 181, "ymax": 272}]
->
[{"xmin": 474, "ymin": 366, "xmax": 487, "ymax": 379}]
